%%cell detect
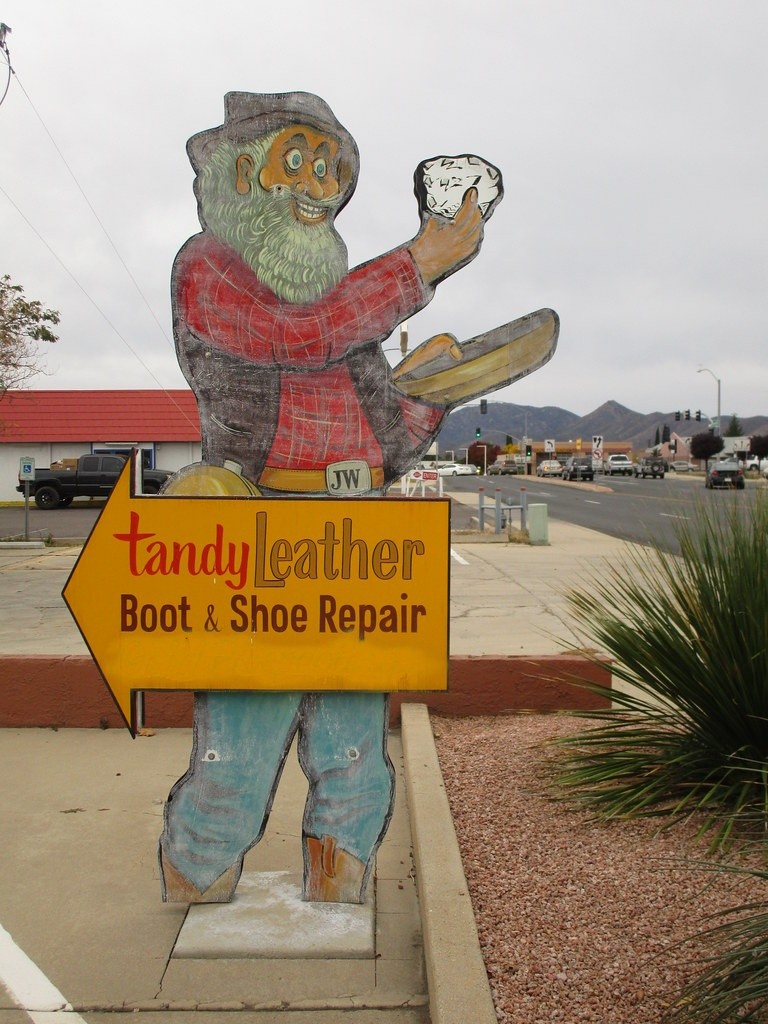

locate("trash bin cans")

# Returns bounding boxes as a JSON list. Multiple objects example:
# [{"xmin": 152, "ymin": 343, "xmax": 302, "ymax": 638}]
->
[{"xmin": 527, "ymin": 503, "xmax": 550, "ymax": 544}]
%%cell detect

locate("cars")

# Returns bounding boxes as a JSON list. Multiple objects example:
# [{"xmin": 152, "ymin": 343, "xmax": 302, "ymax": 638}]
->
[
  {"xmin": 705, "ymin": 450, "xmax": 768, "ymax": 489},
  {"xmin": 560, "ymin": 455, "xmax": 595, "ymax": 481},
  {"xmin": 486, "ymin": 459, "xmax": 518, "ymax": 476},
  {"xmin": 435, "ymin": 462, "xmax": 471, "ymax": 476},
  {"xmin": 536, "ymin": 458, "xmax": 563, "ymax": 478},
  {"xmin": 669, "ymin": 460, "xmax": 700, "ymax": 473}
]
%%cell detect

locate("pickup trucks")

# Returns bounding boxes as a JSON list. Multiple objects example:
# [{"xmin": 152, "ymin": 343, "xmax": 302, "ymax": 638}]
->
[
  {"xmin": 603, "ymin": 454, "xmax": 634, "ymax": 476},
  {"xmin": 15, "ymin": 450, "xmax": 176, "ymax": 510}
]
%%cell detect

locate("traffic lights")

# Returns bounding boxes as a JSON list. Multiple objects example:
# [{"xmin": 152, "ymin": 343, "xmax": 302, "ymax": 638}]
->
[
  {"xmin": 476, "ymin": 427, "xmax": 480, "ymax": 438},
  {"xmin": 526, "ymin": 445, "xmax": 532, "ymax": 456}
]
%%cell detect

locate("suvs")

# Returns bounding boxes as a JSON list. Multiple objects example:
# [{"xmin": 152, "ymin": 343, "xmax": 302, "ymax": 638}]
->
[{"xmin": 634, "ymin": 456, "xmax": 666, "ymax": 479}]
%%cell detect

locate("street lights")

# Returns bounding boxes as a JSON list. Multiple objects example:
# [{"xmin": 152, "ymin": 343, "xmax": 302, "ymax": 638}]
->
[
  {"xmin": 476, "ymin": 445, "xmax": 487, "ymax": 476},
  {"xmin": 445, "ymin": 450, "xmax": 454, "ymax": 462},
  {"xmin": 696, "ymin": 368, "xmax": 721, "ymax": 435},
  {"xmin": 458, "ymin": 447, "xmax": 468, "ymax": 464}
]
